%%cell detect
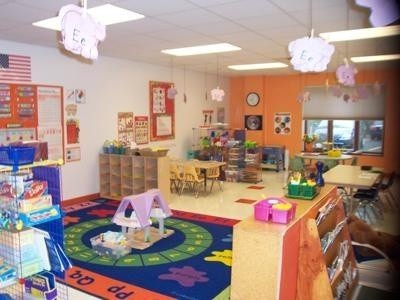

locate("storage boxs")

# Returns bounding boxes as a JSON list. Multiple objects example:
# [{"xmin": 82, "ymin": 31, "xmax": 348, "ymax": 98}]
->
[{"xmin": 224, "ymin": 148, "xmax": 261, "ymax": 183}]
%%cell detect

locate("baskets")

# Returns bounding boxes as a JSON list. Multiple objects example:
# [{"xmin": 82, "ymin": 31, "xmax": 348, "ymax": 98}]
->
[{"xmin": 0, "ymin": 145, "xmax": 34, "ymax": 166}]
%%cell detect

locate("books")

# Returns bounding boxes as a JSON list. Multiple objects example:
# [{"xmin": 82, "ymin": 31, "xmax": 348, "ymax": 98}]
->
[{"xmin": 315, "ymin": 193, "xmax": 363, "ymax": 299}]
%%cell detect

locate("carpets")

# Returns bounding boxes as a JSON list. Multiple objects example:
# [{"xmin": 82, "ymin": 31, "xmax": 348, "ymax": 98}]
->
[{"xmin": 55, "ymin": 192, "xmax": 243, "ymax": 300}]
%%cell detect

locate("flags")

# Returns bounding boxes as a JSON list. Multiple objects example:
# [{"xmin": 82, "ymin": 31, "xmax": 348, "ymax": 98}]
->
[{"xmin": 0, "ymin": 52, "xmax": 32, "ymax": 84}]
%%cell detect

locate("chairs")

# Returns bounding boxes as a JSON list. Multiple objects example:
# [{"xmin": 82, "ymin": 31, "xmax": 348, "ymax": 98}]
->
[
  {"xmin": 353, "ymin": 166, "xmax": 398, "ymax": 225},
  {"xmin": 170, "ymin": 158, "xmax": 226, "ymax": 199}
]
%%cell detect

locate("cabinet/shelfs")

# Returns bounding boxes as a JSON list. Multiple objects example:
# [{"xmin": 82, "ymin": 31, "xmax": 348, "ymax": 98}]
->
[
  {"xmin": 296, "ymin": 196, "xmax": 362, "ymax": 300},
  {"xmin": 98, "ymin": 153, "xmax": 170, "ymax": 206}
]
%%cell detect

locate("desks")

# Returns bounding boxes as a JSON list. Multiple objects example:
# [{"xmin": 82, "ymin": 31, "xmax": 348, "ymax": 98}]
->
[
  {"xmin": 322, "ymin": 164, "xmax": 379, "ymax": 216},
  {"xmin": 295, "ymin": 152, "xmax": 353, "ymax": 167}
]
